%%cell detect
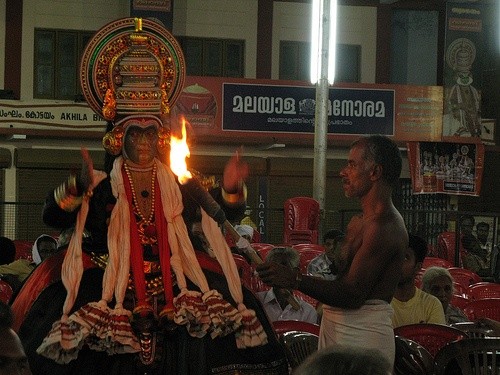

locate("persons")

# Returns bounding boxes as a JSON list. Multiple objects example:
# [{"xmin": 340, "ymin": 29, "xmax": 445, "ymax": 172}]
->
[
  {"xmin": 450, "ymin": 73, "xmax": 483, "ymax": 137},
  {"xmin": 0, "ymin": 111, "xmax": 500, "ymax": 375}
]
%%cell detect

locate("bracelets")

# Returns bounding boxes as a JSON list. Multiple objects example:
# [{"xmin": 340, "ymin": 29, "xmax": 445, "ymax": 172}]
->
[{"xmin": 292, "ymin": 267, "xmax": 303, "ymax": 290}]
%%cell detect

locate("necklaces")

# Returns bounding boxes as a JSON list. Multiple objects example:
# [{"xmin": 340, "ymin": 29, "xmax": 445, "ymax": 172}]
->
[{"xmin": 123, "ymin": 161, "xmax": 158, "ymax": 237}]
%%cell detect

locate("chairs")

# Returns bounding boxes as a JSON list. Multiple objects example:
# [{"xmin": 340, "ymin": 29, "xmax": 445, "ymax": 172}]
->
[{"xmin": 0, "ymin": 195, "xmax": 500, "ymax": 375}]
